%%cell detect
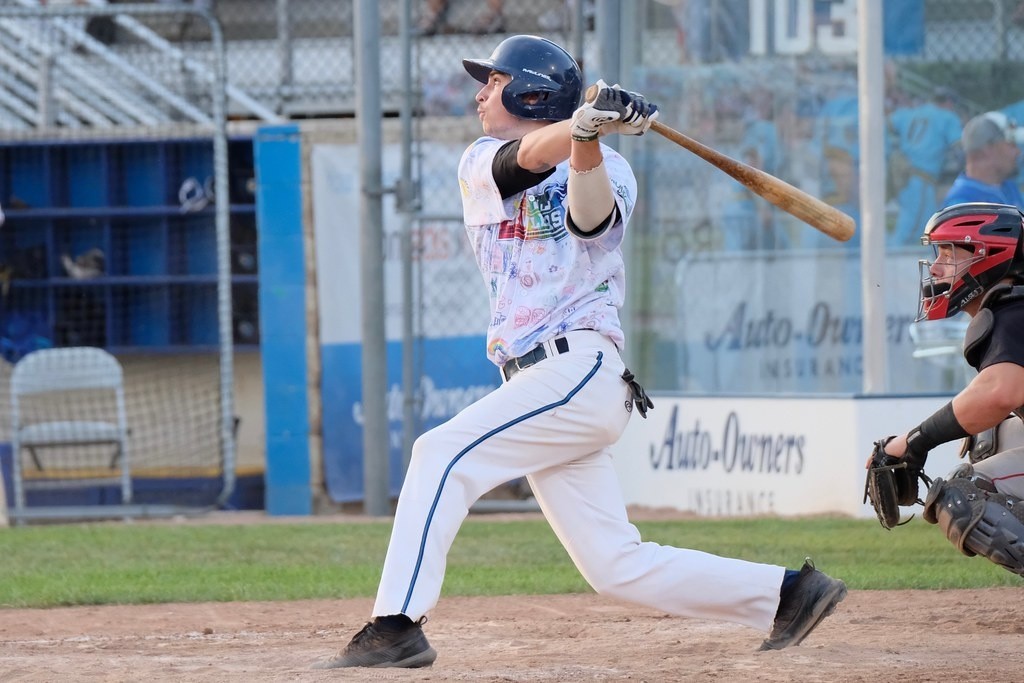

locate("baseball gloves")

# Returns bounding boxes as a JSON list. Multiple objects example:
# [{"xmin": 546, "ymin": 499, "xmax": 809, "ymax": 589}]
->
[{"xmin": 866, "ymin": 435, "xmax": 919, "ymax": 529}]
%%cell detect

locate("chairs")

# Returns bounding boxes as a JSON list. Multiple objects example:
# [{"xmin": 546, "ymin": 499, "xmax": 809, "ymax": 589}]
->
[{"xmin": 11, "ymin": 345, "xmax": 136, "ymax": 526}]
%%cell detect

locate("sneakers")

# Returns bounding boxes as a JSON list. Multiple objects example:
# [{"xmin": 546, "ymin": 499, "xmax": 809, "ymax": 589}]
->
[
  {"xmin": 754, "ymin": 557, "xmax": 848, "ymax": 652},
  {"xmin": 307, "ymin": 615, "xmax": 437, "ymax": 669}
]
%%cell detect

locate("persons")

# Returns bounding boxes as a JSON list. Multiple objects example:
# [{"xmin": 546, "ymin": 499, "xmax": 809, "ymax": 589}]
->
[
  {"xmin": 646, "ymin": 55, "xmax": 1024, "ymax": 247},
  {"xmin": 864, "ymin": 202, "xmax": 1023, "ymax": 574},
  {"xmin": 311, "ymin": 32, "xmax": 849, "ymax": 668},
  {"xmin": 940, "ymin": 110, "xmax": 1021, "ymax": 209}
]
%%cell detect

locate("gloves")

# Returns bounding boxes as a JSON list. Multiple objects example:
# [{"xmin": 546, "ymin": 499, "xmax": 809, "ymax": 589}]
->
[
  {"xmin": 570, "ymin": 84, "xmax": 631, "ymax": 141},
  {"xmin": 621, "ymin": 367, "xmax": 654, "ymax": 419},
  {"xmin": 600, "ymin": 90, "xmax": 660, "ymax": 136}
]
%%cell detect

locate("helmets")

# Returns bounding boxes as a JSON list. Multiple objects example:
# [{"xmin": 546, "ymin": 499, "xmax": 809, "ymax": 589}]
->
[
  {"xmin": 914, "ymin": 202, "xmax": 1022, "ymax": 323},
  {"xmin": 462, "ymin": 34, "xmax": 582, "ymax": 121}
]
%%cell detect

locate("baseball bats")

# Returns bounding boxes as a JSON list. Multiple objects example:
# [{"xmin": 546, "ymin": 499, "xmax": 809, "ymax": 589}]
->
[{"xmin": 583, "ymin": 85, "xmax": 857, "ymax": 243}]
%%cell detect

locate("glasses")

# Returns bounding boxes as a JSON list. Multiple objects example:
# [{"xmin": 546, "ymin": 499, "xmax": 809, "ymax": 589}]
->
[{"xmin": 993, "ymin": 118, "xmax": 1018, "ymax": 142}]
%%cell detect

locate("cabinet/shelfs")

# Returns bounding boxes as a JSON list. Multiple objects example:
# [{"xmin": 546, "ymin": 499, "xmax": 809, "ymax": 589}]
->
[{"xmin": 0, "ymin": 135, "xmax": 264, "ymax": 358}]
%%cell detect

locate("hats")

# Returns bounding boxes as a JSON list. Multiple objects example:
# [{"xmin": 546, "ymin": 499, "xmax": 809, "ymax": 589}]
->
[{"xmin": 961, "ymin": 110, "xmax": 1024, "ymax": 149}]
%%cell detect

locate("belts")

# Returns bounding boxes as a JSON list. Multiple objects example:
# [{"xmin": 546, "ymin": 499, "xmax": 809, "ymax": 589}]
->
[{"xmin": 503, "ymin": 337, "xmax": 569, "ymax": 382}]
